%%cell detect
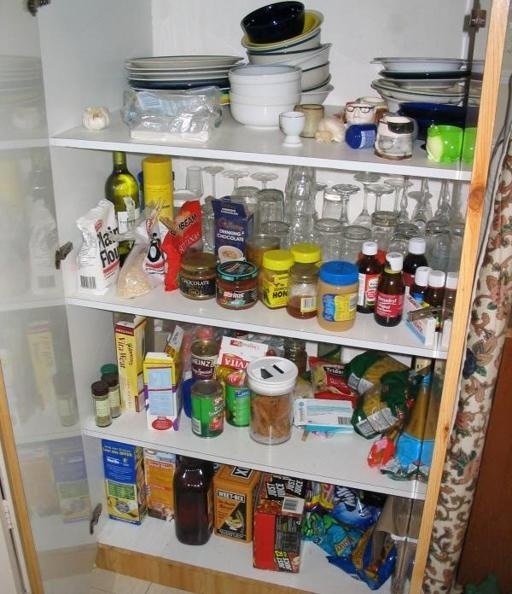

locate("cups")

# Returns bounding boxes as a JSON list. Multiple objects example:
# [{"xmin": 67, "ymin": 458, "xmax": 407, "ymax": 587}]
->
[
  {"xmin": 347, "ymin": 96, "xmax": 462, "ymax": 163},
  {"xmin": 186, "ymin": 166, "xmax": 203, "ymax": 197}
]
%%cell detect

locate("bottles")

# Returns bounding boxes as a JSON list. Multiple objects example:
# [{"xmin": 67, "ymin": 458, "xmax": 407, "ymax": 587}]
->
[
  {"xmin": 173, "ymin": 189, "xmax": 199, "ymax": 216},
  {"xmin": 52, "ymin": 365, "xmax": 77, "ymax": 427},
  {"xmin": 106, "ymin": 152, "xmax": 139, "ymax": 255},
  {"xmin": 92, "ymin": 362, "xmax": 122, "ymax": 428},
  {"xmin": 245, "ymin": 356, "xmax": 299, "ymax": 446},
  {"xmin": 142, "ymin": 156, "xmax": 174, "ymax": 222},
  {"xmin": 357, "ymin": 233, "xmax": 459, "ymax": 327},
  {"xmin": 26, "ymin": 149, "xmax": 58, "ymax": 246},
  {"xmin": 173, "ymin": 454, "xmax": 215, "ymax": 547}
]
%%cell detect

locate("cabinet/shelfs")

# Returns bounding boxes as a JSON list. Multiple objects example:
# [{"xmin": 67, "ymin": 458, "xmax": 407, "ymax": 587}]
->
[{"xmin": 1, "ymin": 1, "xmax": 512, "ymax": 594}]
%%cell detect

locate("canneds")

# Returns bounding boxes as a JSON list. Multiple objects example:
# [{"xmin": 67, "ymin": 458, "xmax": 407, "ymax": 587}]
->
[
  {"xmin": 179, "ymin": 251, "xmax": 219, "ymax": 300},
  {"xmin": 215, "ymin": 261, "xmax": 258, "ymax": 309},
  {"xmin": 190, "ymin": 339, "xmax": 221, "ymax": 380},
  {"xmin": 190, "ymin": 379, "xmax": 224, "ymax": 437},
  {"xmin": 259, "ymin": 242, "xmax": 358, "ymax": 331},
  {"xmin": 225, "ymin": 384, "xmax": 256, "ymax": 427}
]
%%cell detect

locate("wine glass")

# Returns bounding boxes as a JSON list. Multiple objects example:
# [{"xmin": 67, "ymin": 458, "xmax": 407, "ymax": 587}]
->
[{"xmin": 199, "ymin": 165, "xmax": 466, "ymax": 272}]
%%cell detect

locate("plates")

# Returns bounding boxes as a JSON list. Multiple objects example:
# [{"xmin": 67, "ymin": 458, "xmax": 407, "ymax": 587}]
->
[
  {"xmin": 467, "ymin": 60, "xmax": 485, "ymax": 108},
  {"xmin": 123, "ymin": 56, "xmax": 241, "ymax": 107},
  {"xmin": 369, "ymin": 55, "xmax": 469, "ymax": 138},
  {"xmin": 0, "ymin": 53, "xmax": 43, "ymax": 112}
]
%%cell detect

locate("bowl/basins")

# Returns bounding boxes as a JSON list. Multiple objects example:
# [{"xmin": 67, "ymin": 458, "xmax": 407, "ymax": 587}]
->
[{"xmin": 227, "ymin": 0, "xmax": 333, "ymax": 146}]
